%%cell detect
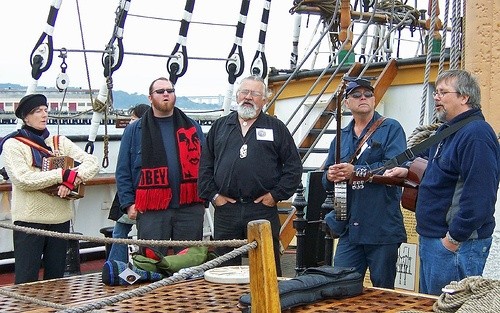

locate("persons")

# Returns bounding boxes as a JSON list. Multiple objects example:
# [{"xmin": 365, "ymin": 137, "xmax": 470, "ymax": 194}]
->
[
  {"xmin": 197, "ymin": 74, "xmax": 304, "ymax": 278},
  {"xmin": 107, "ymin": 104, "xmax": 151, "ymax": 263},
  {"xmin": 382, "ymin": 70, "xmax": 499, "ymax": 298},
  {"xmin": 0, "ymin": 94, "xmax": 101, "ymax": 286},
  {"xmin": 114, "ymin": 76, "xmax": 205, "ymax": 260},
  {"xmin": 320, "ymin": 78, "xmax": 408, "ymax": 291}
]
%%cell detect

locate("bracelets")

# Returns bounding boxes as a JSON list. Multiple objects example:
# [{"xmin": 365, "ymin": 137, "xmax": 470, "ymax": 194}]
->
[{"xmin": 445, "ymin": 231, "xmax": 461, "ymax": 246}]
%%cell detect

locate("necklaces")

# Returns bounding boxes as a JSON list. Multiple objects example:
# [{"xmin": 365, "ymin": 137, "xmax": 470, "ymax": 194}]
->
[
  {"xmin": 235, "ymin": 124, "xmax": 257, "ymax": 159},
  {"xmin": 239, "ymin": 116, "xmax": 251, "ymax": 127}
]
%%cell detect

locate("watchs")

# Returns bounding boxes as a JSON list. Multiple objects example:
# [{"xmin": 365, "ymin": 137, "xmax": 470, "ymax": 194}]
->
[{"xmin": 211, "ymin": 193, "xmax": 220, "ymax": 204}]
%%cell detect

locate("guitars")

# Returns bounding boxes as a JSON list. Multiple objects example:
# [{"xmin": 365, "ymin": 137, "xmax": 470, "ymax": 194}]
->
[
  {"xmin": 349, "ymin": 157, "xmax": 428, "ymax": 212},
  {"xmin": 333, "ymin": 79, "xmax": 348, "ymax": 222}
]
